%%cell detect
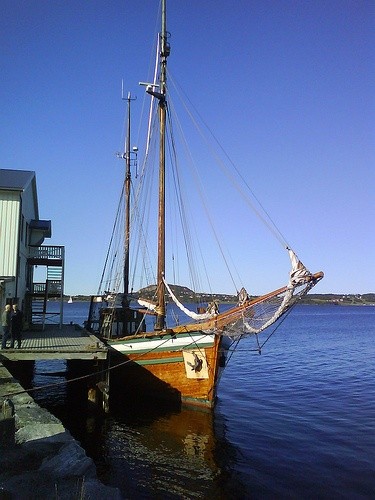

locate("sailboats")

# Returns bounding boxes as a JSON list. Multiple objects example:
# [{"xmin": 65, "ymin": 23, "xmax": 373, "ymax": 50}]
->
[{"xmin": 86, "ymin": 1, "xmax": 322, "ymax": 407}]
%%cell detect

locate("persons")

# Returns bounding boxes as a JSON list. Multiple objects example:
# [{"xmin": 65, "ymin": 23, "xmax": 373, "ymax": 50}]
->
[{"xmin": 1, "ymin": 305, "xmax": 25, "ymax": 350}]
